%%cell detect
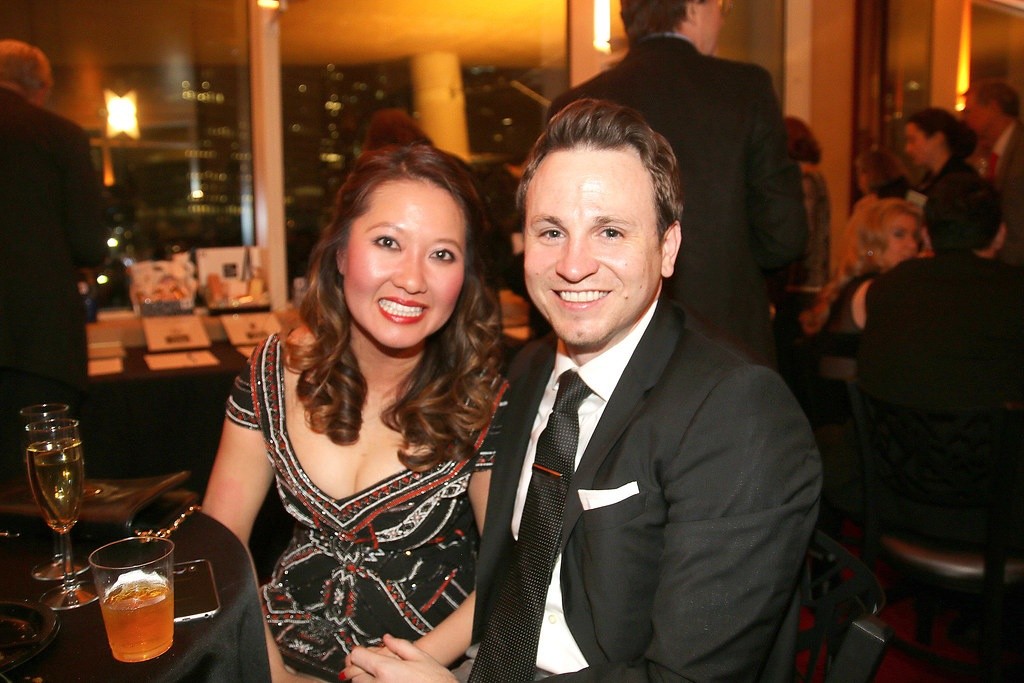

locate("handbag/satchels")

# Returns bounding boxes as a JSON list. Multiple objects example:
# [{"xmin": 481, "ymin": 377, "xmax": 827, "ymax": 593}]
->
[{"xmin": 0, "ymin": 471, "xmax": 200, "ymax": 542}]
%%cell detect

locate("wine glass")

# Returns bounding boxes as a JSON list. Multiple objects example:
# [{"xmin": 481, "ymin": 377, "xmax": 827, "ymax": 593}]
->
[
  {"xmin": 25, "ymin": 418, "xmax": 100, "ymax": 610},
  {"xmin": 20, "ymin": 404, "xmax": 90, "ymax": 580}
]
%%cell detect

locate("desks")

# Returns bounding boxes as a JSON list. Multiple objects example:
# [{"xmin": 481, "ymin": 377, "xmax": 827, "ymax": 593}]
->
[{"xmin": 0, "ymin": 490, "xmax": 271, "ymax": 683}]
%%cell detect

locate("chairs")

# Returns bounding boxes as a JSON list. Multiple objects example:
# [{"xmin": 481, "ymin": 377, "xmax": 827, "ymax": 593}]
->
[
  {"xmin": 850, "ymin": 383, "xmax": 1024, "ymax": 683},
  {"xmin": 789, "ymin": 528, "xmax": 897, "ymax": 683}
]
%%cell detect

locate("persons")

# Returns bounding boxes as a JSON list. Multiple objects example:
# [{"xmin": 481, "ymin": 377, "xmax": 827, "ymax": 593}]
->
[
  {"xmin": 961, "ymin": 79, "xmax": 1023, "ymax": 267},
  {"xmin": 0, "ymin": 37, "xmax": 110, "ymax": 471},
  {"xmin": 842, "ymin": 147, "xmax": 927, "ymax": 268},
  {"xmin": 855, "ymin": 168, "xmax": 1024, "ymax": 560},
  {"xmin": 346, "ymin": 98, "xmax": 826, "ymax": 683},
  {"xmin": 545, "ymin": 0, "xmax": 810, "ymax": 379},
  {"xmin": 198, "ymin": 145, "xmax": 513, "ymax": 683},
  {"xmin": 782, "ymin": 113, "xmax": 831, "ymax": 285},
  {"xmin": 363, "ymin": 109, "xmax": 495, "ymax": 250},
  {"xmin": 790, "ymin": 192, "xmax": 924, "ymax": 514},
  {"xmin": 904, "ymin": 106, "xmax": 983, "ymax": 192}
]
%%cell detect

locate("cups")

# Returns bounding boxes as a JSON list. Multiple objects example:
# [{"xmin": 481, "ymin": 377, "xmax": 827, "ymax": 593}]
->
[{"xmin": 88, "ymin": 537, "xmax": 175, "ymax": 662}]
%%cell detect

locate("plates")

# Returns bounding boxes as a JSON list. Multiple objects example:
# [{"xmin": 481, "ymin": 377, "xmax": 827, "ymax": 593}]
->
[{"xmin": 0, "ymin": 597, "xmax": 63, "ymax": 674}]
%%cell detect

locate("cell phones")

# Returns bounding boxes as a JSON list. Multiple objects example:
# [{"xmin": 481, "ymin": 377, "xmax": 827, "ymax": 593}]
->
[{"xmin": 172, "ymin": 559, "xmax": 222, "ymax": 622}]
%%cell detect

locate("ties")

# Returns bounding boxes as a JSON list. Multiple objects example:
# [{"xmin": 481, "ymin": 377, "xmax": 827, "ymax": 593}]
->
[
  {"xmin": 466, "ymin": 369, "xmax": 594, "ymax": 683},
  {"xmin": 986, "ymin": 153, "xmax": 997, "ymax": 180}
]
